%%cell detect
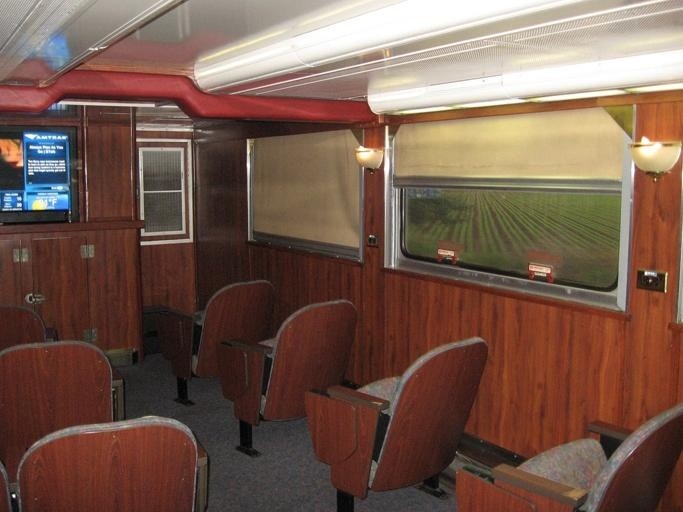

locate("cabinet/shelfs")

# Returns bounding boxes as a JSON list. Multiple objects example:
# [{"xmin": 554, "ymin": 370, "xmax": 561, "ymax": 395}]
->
[{"xmin": 0, "ymin": 105, "xmax": 146, "ymax": 368}]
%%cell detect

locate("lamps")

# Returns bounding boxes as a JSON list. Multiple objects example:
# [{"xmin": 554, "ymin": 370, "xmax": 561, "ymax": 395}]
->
[
  {"xmin": 366, "ymin": 48, "xmax": 683, "ymax": 117},
  {"xmin": 626, "ymin": 141, "xmax": 682, "ymax": 184}
]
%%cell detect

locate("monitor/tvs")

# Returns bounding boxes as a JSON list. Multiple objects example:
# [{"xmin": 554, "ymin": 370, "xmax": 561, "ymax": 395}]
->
[{"xmin": 0, "ymin": 125, "xmax": 80, "ymax": 225}]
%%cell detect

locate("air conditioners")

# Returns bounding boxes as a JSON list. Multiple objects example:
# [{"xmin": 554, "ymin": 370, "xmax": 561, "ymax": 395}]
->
[{"xmin": 354, "ymin": 146, "xmax": 385, "ymax": 176}]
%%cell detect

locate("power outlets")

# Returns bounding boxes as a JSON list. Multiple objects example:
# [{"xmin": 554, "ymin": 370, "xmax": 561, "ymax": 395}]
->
[{"xmin": 636, "ymin": 270, "xmax": 668, "ymax": 293}]
